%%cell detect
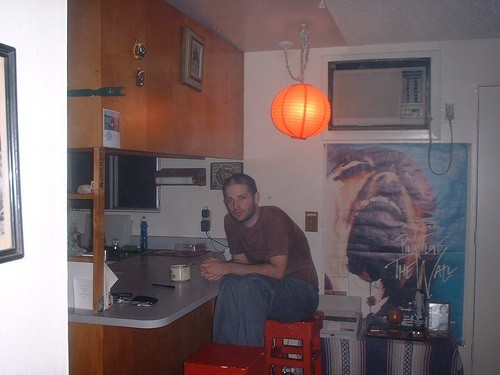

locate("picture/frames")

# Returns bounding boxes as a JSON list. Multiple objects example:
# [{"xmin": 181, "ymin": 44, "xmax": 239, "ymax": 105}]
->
[
  {"xmin": 0, "ymin": 43, "xmax": 24, "ymax": 263},
  {"xmin": 426, "ymin": 301, "xmax": 450, "ymax": 332},
  {"xmin": 182, "ymin": 25, "xmax": 205, "ymax": 91}
]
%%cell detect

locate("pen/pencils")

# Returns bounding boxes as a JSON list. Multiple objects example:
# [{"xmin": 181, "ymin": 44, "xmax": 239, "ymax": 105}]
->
[{"xmin": 157, "ymin": 285, "xmax": 175, "ymax": 288}]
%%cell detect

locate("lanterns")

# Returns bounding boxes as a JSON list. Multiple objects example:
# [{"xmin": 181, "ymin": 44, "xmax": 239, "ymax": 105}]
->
[{"xmin": 271, "ymin": 84, "xmax": 332, "ymax": 140}]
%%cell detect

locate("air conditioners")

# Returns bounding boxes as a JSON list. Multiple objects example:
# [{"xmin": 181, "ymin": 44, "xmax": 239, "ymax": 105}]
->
[{"xmin": 331, "ymin": 68, "xmax": 426, "ymax": 127}]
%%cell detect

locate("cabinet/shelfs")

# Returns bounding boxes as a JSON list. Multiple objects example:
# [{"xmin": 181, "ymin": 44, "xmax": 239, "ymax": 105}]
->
[{"xmin": 67, "ymin": 0, "xmax": 244, "ymax": 161}]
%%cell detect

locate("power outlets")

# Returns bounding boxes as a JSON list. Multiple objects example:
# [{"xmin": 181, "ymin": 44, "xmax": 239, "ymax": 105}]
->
[
  {"xmin": 202, "ymin": 212, "xmax": 211, "ymax": 231},
  {"xmin": 444, "ymin": 103, "xmax": 454, "ymax": 119}
]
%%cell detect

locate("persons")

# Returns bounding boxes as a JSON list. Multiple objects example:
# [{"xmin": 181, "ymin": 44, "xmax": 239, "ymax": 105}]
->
[{"xmin": 200, "ymin": 173, "xmax": 319, "ymax": 347}]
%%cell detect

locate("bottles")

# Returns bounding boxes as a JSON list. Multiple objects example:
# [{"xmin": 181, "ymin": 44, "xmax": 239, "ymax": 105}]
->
[{"xmin": 72, "ymin": 223, "xmax": 81, "ymax": 246}]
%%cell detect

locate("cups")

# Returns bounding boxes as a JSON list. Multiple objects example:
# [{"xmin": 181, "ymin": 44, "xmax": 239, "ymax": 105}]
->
[{"xmin": 169, "ymin": 265, "xmax": 191, "ymax": 282}]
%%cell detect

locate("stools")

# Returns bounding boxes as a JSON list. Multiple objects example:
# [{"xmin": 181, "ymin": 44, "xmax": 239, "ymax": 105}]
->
[{"xmin": 183, "ymin": 311, "xmax": 323, "ymax": 375}]
